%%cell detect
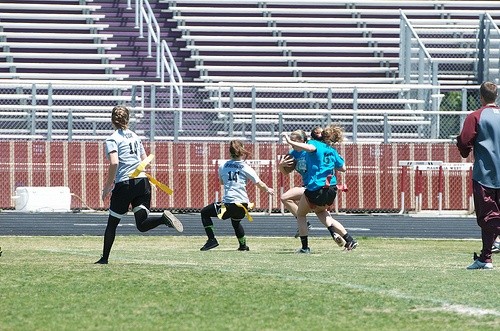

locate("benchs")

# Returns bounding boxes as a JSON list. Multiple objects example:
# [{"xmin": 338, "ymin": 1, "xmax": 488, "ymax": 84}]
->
[{"xmin": 0, "ymin": 0, "xmax": 500, "ymax": 163}]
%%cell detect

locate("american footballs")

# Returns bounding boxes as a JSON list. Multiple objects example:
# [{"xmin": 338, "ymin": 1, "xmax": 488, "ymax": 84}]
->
[{"xmin": 284, "ymin": 153, "xmax": 297, "ymax": 173}]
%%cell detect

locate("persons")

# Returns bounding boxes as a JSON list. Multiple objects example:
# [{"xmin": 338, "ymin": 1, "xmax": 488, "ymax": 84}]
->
[
  {"xmin": 94, "ymin": 105, "xmax": 184, "ymax": 266},
  {"xmin": 278, "ymin": 123, "xmax": 358, "ymax": 254},
  {"xmin": 456, "ymin": 82, "xmax": 500, "ymax": 269},
  {"xmin": 200, "ymin": 140, "xmax": 275, "ymax": 251}
]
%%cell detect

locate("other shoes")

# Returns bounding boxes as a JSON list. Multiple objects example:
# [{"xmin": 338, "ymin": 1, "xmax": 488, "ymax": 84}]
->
[
  {"xmin": 237, "ymin": 246, "xmax": 250, "ymax": 252},
  {"xmin": 465, "ymin": 260, "xmax": 493, "ymax": 271},
  {"xmin": 295, "ymin": 221, "xmax": 312, "ymax": 238},
  {"xmin": 343, "ymin": 240, "xmax": 358, "ymax": 252},
  {"xmin": 94, "ymin": 257, "xmax": 108, "ymax": 265},
  {"xmin": 200, "ymin": 238, "xmax": 219, "ymax": 251},
  {"xmin": 295, "ymin": 248, "xmax": 311, "ymax": 255},
  {"xmin": 491, "ymin": 241, "xmax": 500, "ymax": 253},
  {"xmin": 162, "ymin": 209, "xmax": 184, "ymax": 233},
  {"xmin": 333, "ymin": 232, "xmax": 345, "ymax": 247}
]
